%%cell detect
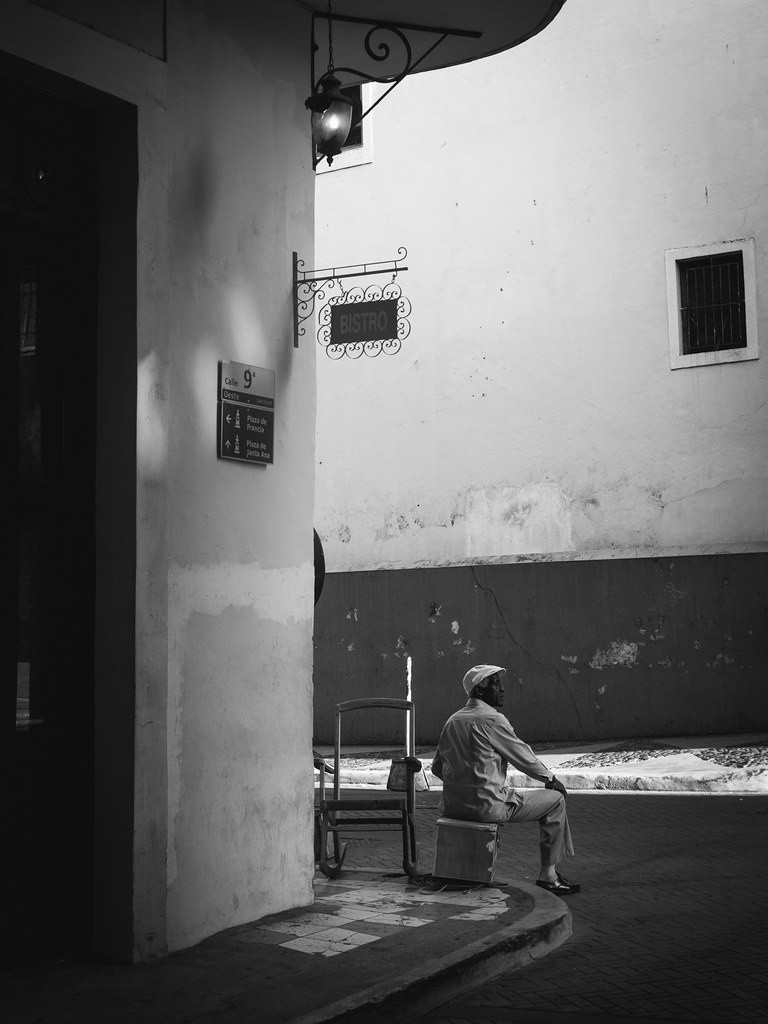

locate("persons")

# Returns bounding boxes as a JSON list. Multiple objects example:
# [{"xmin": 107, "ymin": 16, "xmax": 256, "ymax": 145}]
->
[{"xmin": 432, "ymin": 665, "xmax": 582, "ymax": 894}]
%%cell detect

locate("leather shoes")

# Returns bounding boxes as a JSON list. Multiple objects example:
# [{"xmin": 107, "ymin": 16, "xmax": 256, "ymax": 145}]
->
[{"xmin": 537, "ymin": 870, "xmax": 579, "ymax": 894}]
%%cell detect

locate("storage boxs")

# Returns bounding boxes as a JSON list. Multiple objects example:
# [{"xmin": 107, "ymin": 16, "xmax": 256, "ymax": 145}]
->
[{"xmin": 431, "ymin": 818, "xmax": 500, "ymax": 884}]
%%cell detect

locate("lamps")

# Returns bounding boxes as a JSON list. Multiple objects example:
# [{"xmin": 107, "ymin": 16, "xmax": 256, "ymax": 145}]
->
[{"xmin": 304, "ymin": 24, "xmax": 412, "ymax": 167}]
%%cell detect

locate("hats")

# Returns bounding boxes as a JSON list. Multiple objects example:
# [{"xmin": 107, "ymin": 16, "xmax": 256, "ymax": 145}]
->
[{"xmin": 462, "ymin": 664, "xmax": 507, "ymax": 695}]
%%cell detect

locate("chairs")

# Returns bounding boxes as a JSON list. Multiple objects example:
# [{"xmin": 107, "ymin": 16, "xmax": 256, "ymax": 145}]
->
[{"xmin": 314, "ymin": 697, "xmax": 419, "ymax": 877}]
